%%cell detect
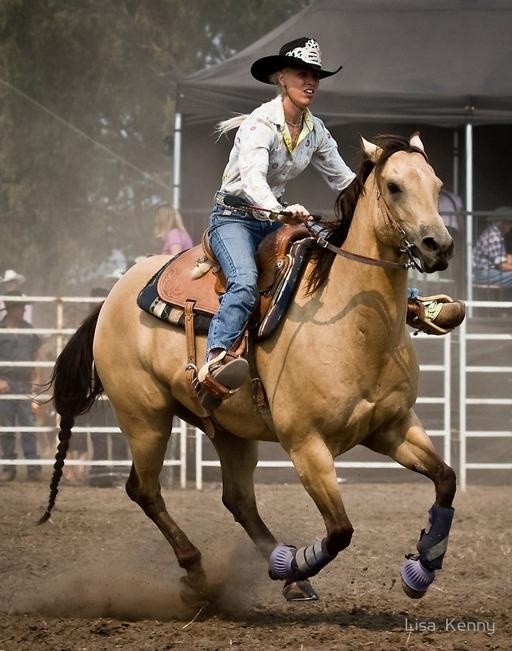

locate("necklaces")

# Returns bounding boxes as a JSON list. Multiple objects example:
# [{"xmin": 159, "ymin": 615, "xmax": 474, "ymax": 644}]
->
[{"xmin": 285, "ymin": 112, "xmax": 303, "ymax": 127}]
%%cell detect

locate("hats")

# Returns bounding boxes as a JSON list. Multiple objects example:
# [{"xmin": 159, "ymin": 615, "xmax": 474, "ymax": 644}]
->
[
  {"xmin": 250, "ymin": 39, "xmax": 343, "ymax": 85},
  {"xmin": 487, "ymin": 206, "xmax": 512, "ymax": 221},
  {"xmin": 1, "ymin": 269, "xmax": 27, "ymax": 285},
  {"xmin": 0, "ymin": 289, "xmax": 33, "ymax": 311}
]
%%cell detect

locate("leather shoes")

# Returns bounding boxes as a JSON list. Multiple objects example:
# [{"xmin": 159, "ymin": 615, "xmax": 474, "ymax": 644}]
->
[
  {"xmin": 408, "ymin": 300, "xmax": 466, "ymax": 335},
  {"xmin": 200, "ymin": 358, "xmax": 250, "ymax": 412}
]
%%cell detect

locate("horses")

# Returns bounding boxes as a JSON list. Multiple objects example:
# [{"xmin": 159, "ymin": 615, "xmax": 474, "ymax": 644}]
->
[{"xmin": 26, "ymin": 131, "xmax": 458, "ymax": 607}]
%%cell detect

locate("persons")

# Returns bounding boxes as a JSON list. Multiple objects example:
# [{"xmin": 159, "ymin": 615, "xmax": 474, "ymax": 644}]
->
[
  {"xmin": 194, "ymin": 35, "xmax": 466, "ymax": 412},
  {"xmin": 152, "ymin": 204, "xmax": 192, "ymax": 256},
  {"xmin": 437, "ymin": 188, "xmax": 465, "ymax": 237},
  {"xmin": 0, "ymin": 269, "xmax": 129, "ymax": 483},
  {"xmin": 471, "ymin": 206, "xmax": 512, "ymax": 287}
]
